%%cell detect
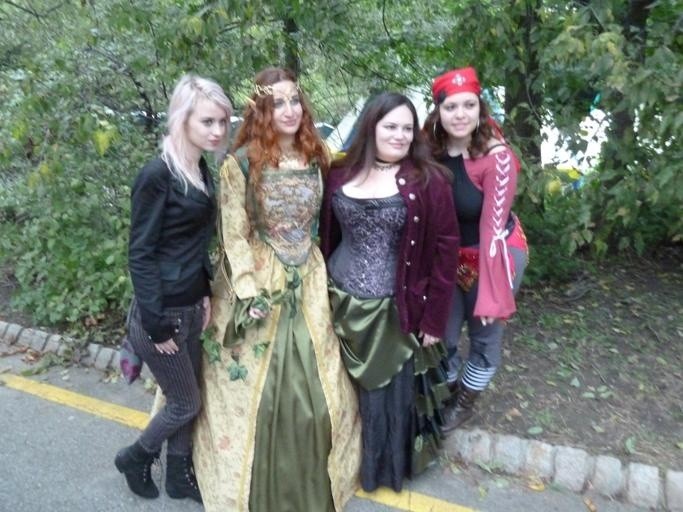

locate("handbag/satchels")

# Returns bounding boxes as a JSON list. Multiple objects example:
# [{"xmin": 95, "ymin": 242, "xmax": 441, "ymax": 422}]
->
[{"xmin": 454, "ymin": 212, "xmax": 529, "ymax": 298}]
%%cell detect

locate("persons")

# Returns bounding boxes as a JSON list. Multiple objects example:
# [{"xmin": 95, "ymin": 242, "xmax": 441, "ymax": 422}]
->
[
  {"xmin": 112, "ymin": 73, "xmax": 234, "ymax": 504},
  {"xmin": 193, "ymin": 65, "xmax": 362, "ymax": 511},
  {"xmin": 416, "ymin": 65, "xmax": 531, "ymax": 435},
  {"xmin": 318, "ymin": 90, "xmax": 459, "ymax": 494}
]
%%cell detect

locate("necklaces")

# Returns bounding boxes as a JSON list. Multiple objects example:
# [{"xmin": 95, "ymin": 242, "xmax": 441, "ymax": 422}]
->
[
  {"xmin": 275, "ymin": 149, "xmax": 303, "ymax": 170},
  {"xmin": 369, "ymin": 155, "xmax": 405, "ymax": 175}
]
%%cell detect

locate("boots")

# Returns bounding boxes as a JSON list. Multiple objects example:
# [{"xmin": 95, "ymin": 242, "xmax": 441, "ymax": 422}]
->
[
  {"xmin": 438, "ymin": 383, "xmax": 480, "ymax": 432},
  {"xmin": 115, "ymin": 441, "xmax": 161, "ymax": 499},
  {"xmin": 166, "ymin": 452, "xmax": 203, "ymax": 503}
]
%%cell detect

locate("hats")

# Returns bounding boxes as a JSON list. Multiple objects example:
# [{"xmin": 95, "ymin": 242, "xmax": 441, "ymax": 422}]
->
[{"xmin": 431, "ymin": 66, "xmax": 482, "ymax": 104}]
